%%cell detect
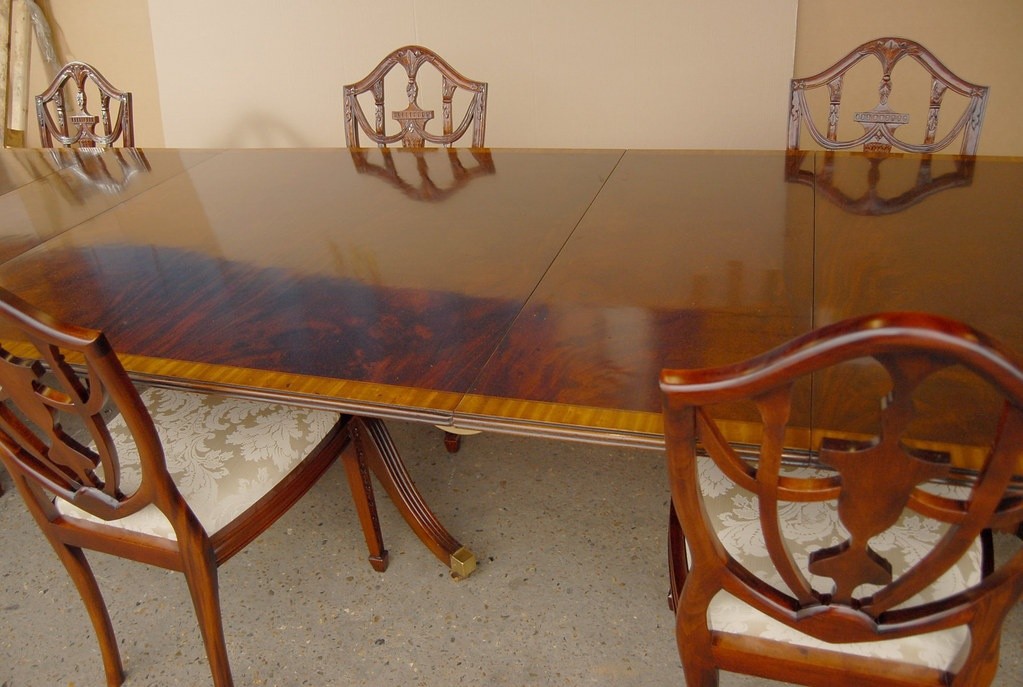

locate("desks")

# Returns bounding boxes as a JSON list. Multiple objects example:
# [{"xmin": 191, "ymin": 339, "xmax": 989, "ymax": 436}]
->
[{"xmin": 0, "ymin": 147, "xmax": 1023, "ymax": 581}]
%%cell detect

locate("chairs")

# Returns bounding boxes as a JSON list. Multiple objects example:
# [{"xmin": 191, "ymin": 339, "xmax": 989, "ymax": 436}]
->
[
  {"xmin": 35, "ymin": 61, "xmax": 135, "ymax": 147},
  {"xmin": 344, "ymin": 45, "xmax": 489, "ymax": 146},
  {"xmin": 46, "ymin": 147, "xmax": 152, "ymax": 194},
  {"xmin": 658, "ymin": 310, "xmax": 1023, "ymax": 687},
  {"xmin": 785, "ymin": 149, "xmax": 977, "ymax": 215},
  {"xmin": 786, "ymin": 37, "xmax": 990, "ymax": 154},
  {"xmin": 0, "ymin": 288, "xmax": 391, "ymax": 687},
  {"xmin": 349, "ymin": 147, "xmax": 496, "ymax": 204}
]
